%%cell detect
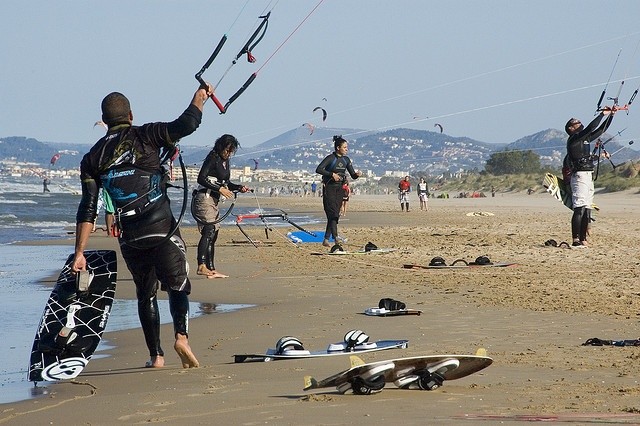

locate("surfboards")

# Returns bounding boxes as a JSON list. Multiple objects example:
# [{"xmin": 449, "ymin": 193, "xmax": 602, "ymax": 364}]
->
[
  {"xmin": 287, "ymin": 230, "xmax": 345, "ymax": 243},
  {"xmin": 366, "ymin": 308, "xmax": 422, "ymax": 316},
  {"xmin": 542, "ymin": 173, "xmax": 599, "ymax": 223},
  {"xmin": 26, "ymin": 250, "xmax": 117, "ymax": 380},
  {"xmin": 403, "ymin": 262, "xmax": 517, "ymax": 269},
  {"xmin": 303, "ymin": 349, "xmax": 493, "ymax": 391},
  {"xmin": 231, "ymin": 339, "xmax": 409, "ymax": 362},
  {"xmin": 311, "ymin": 249, "xmax": 397, "ymax": 256}
]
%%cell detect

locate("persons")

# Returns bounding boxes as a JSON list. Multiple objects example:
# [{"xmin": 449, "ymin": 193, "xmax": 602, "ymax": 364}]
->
[
  {"xmin": 563, "ymin": 149, "xmax": 611, "ymax": 234},
  {"xmin": 71, "ymin": 82, "xmax": 215, "ymax": 370},
  {"xmin": 42, "ymin": 176, "xmax": 53, "ymax": 194},
  {"xmin": 341, "ymin": 181, "xmax": 351, "ymax": 215},
  {"xmin": 187, "ymin": 133, "xmax": 245, "ymax": 284},
  {"xmin": 352, "ymin": 185, "xmax": 394, "ymax": 195},
  {"xmin": 262, "ymin": 180, "xmax": 323, "ymax": 198},
  {"xmin": 397, "ymin": 175, "xmax": 413, "ymax": 213},
  {"xmin": 563, "ymin": 102, "xmax": 620, "ymax": 245},
  {"xmin": 432, "ymin": 191, "xmax": 488, "ymax": 199},
  {"xmin": 490, "ymin": 185, "xmax": 496, "ymax": 198},
  {"xmin": 313, "ymin": 134, "xmax": 361, "ymax": 247},
  {"xmin": 417, "ymin": 176, "xmax": 431, "ymax": 212}
]
